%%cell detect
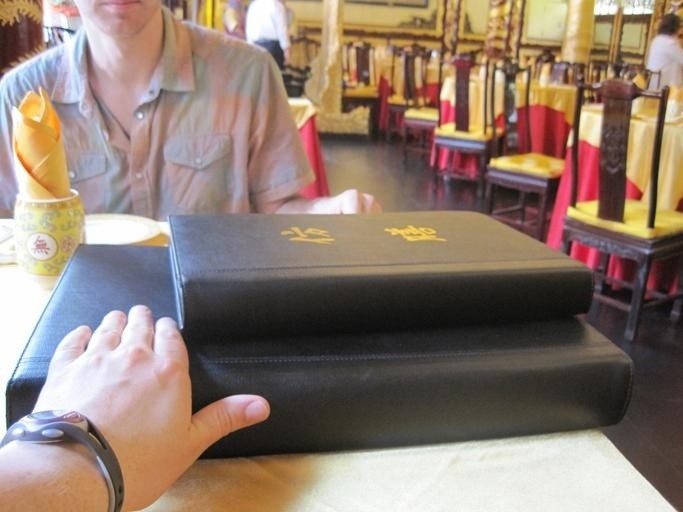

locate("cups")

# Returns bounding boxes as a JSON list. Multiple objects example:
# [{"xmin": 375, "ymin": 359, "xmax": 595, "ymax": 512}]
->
[{"xmin": 13, "ymin": 188, "xmax": 86, "ymax": 277}]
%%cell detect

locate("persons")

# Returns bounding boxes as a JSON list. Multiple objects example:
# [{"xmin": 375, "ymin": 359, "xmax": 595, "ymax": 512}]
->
[
  {"xmin": 245, "ymin": 0, "xmax": 291, "ymax": 94},
  {"xmin": 0, "ymin": 1, "xmax": 383, "ymax": 221},
  {"xmin": 0, "ymin": 304, "xmax": 271, "ymax": 512},
  {"xmin": 646, "ymin": 13, "xmax": 683, "ymax": 95}
]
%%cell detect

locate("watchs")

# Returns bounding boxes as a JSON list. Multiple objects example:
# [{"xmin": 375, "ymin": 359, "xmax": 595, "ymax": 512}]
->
[{"xmin": 0, "ymin": 409, "xmax": 125, "ymax": 512}]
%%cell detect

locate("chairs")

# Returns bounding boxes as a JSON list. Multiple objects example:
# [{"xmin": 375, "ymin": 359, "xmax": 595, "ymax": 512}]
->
[{"xmin": 279, "ymin": 34, "xmax": 683, "ymax": 342}]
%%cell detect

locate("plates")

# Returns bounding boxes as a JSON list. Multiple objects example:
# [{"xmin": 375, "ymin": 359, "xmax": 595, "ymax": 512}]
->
[{"xmin": 86, "ymin": 213, "xmax": 161, "ymax": 244}]
